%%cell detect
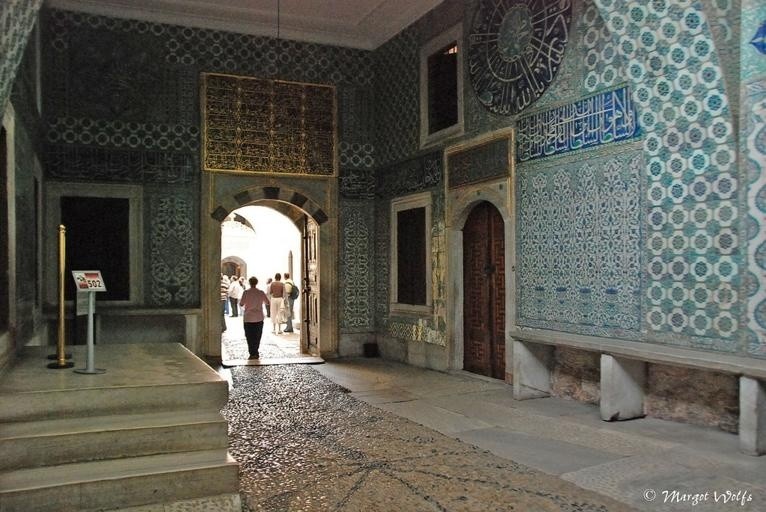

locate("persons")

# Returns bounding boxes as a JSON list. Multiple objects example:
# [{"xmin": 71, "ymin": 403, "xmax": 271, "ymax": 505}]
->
[{"xmin": 220, "ymin": 271, "xmax": 300, "ymax": 360}]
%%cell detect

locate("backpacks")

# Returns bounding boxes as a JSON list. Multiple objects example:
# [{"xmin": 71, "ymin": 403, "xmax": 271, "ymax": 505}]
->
[{"xmin": 285, "ymin": 281, "xmax": 299, "ymax": 299}]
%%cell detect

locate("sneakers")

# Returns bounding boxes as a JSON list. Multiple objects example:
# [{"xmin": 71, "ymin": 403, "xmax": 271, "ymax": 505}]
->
[
  {"xmin": 248, "ymin": 353, "xmax": 259, "ymax": 360},
  {"xmin": 272, "ymin": 327, "xmax": 293, "ymax": 334}
]
